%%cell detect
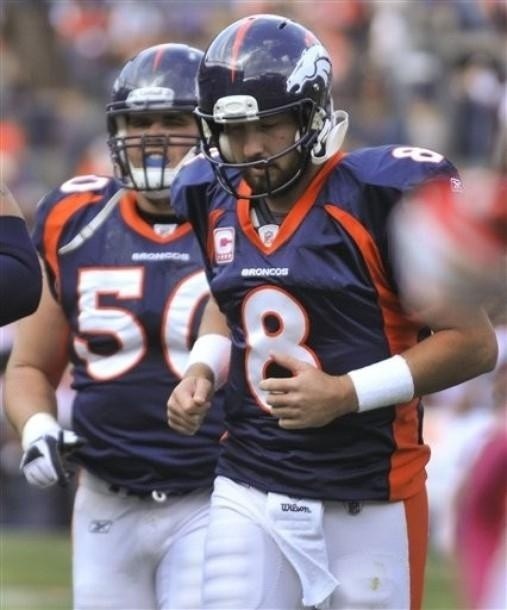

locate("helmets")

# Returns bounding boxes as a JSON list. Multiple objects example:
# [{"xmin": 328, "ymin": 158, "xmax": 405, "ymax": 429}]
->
[
  {"xmin": 195, "ymin": 13, "xmax": 332, "ymax": 200},
  {"xmin": 103, "ymin": 43, "xmax": 207, "ymax": 191}
]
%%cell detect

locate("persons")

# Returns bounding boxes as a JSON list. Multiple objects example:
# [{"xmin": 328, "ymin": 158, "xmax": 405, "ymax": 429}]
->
[
  {"xmin": 0, "ymin": 178, "xmax": 41, "ymax": 327},
  {"xmin": 167, "ymin": 16, "xmax": 498, "ymax": 610},
  {"xmin": 0, "ymin": 0, "xmax": 506, "ymax": 609},
  {"xmin": 0, "ymin": 44, "xmax": 227, "ymax": 609}
]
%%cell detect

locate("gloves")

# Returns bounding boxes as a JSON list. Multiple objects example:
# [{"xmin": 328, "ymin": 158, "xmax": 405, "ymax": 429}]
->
[{"xmin": 19, "ymin": 411, "xmax": 81, "ymax": 488}]
